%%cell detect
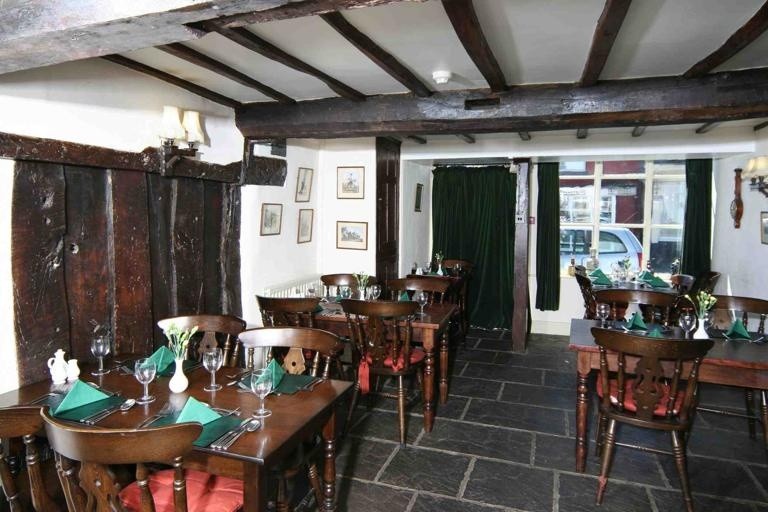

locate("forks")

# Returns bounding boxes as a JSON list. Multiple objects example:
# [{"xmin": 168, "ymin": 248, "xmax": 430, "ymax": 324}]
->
[{"xmin": 295, "ymin": 378, "xmax": 322, "ymax": 392}]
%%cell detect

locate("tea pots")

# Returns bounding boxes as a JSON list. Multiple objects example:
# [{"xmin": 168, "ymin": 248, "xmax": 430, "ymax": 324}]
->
[{"xmin": 47, "ymin": 349, "xmax": 67, "ymax": 386}]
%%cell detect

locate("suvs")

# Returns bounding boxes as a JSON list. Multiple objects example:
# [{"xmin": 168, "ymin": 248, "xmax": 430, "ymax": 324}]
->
[{"xmin": 558, "ymin": 224, "xmax": 643, "ymax": 278}]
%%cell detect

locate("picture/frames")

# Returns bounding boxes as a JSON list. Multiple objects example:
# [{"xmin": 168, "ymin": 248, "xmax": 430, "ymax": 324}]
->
[
  {"xmin": 295, "ymin": 208, "xmax": 314, "ymax": 244},
  {"xmin": 257, "ymin": 202, "xmax": 283, "ymax": 236},
  {"xmin": 293, "ymin": 166, "xmax": 314, "ymax": 203},
  {"xmin": 336, "ymin": 165, "xmax": 365, "ymax": 200},
  {"xmin": 759, "ymin": 209, "xmax": 768, "ymax": 246},
  {"xmin": 335, "ymin": 219, "xmax": 368, "ymax": 251}
]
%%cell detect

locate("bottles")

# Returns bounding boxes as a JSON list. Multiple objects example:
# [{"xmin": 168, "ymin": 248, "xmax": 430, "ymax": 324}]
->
[
  {"xmin": 568, "ymin": 259, "xmax": 576, "ymax": 275},
  {"xmin": 585, "ymin": 248, "xmax": 600, "ymax": 273},
  {"xmin": 68, "ymin": 359, "xmax": 81, "ymax": 380}
]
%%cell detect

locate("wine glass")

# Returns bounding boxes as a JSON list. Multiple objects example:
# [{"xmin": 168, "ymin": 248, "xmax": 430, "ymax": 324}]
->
[
  {"xmin": 593, "ymin": 299, "xmax": 714, "ymax": 342},
  {"xmin": 135, "ymin": 358, "xmax": 154, "ymax": 405},
  {"xmin": 412, "ymin": 262, "xmax": 463, "ymax": 278},
  {"xmin": 307, "ymin": 284, "xmax": 428, "ymax": 317},
  {"xmin": 250, "ymin": 369, "xmax": 274, "ymax": 418},
  {"xmin": 610, "ymin": 261, "xmax": 641, "ymax": 284},
  {"xmin": 90, "ymin": 336, "xmax": 111, "ymax": 376},
  {"xmin": 202, "ymin": 348, "xmax": 224, "ymax": 392}
]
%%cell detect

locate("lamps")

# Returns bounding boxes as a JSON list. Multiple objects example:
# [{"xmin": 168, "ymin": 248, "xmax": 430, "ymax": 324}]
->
[
  {"xmin": 738, "ymin": 153, "xmax": 767, "ymax": 199},
  {"xmin": 141, "ymin": 105, "xmax": 209, "ymax": 177}
]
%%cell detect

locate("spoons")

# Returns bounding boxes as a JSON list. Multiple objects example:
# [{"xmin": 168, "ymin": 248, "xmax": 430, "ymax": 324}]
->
[{"xmin": 78, "ymin": 399, "xmax": 136, "ymax": 425}]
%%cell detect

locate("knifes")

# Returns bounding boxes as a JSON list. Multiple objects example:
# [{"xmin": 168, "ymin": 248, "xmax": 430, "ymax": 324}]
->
[
  {"xmin": 226, "ymin": 368, "xmax": 251, "ymax": 388},
  {"xmin": 211, "ymin": 416, "xmax": 261, "ymax": 453}
]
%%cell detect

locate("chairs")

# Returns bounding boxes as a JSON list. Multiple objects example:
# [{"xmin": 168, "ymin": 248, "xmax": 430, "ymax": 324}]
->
[
  {"xmin": 255, "ymin": 258, "xmax": 477, "ymax": 450},
  {"xmin": 560, "ymin": 259, "xmax": 767, "ymax": 512},
  {"xmin": 2, "ymin": 314, "xmax": 346, "ymax": 511}
]
